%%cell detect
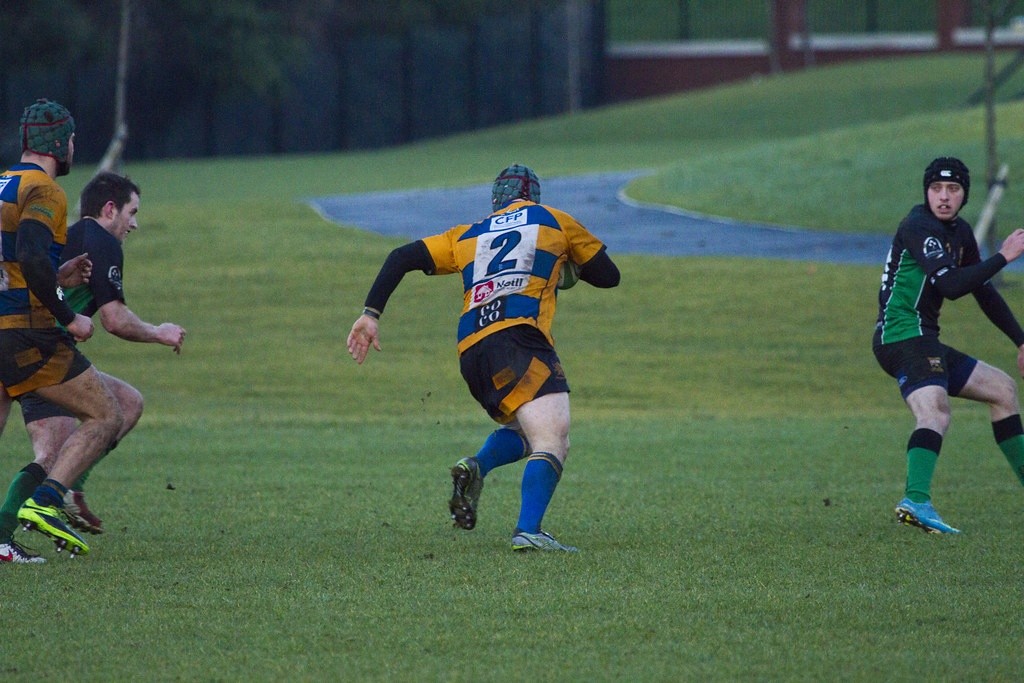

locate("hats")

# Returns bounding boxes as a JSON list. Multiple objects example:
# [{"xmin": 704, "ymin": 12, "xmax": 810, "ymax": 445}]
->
[
  {"xmin": 925, "ymin": 158, "xmax": 970, "ymax": 211},
  {"xmin": 20, "ymin": 98, "xmax": 75, "ymax": 167},
  {"xmin": 492, "ymin": 163, "xmax": 540, "ymax": 211}
]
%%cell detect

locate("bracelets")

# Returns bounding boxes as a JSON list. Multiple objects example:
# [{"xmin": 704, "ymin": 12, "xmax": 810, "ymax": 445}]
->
[{"xmin": 363, "ymin": 308, "xmax": 381, "ymax": 320}]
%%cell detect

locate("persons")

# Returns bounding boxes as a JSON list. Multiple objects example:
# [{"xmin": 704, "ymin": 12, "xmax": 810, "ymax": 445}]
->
[
  {"xmin": 345, "ymin": 161, "xmax": 622, "ymax": 553},
  {"xmin": 872, "ymin": 157, "xmax": 1024, "ymax": 537},
  {"xmin": 0, "ymin": 97, "xmax": 124, "ymax": 559},
  {"xmin": 0, "ymin": 172, "xmax": 186, "ymax": 564}
]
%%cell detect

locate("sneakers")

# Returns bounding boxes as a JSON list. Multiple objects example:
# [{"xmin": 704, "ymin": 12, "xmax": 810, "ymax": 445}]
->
[
  {"xmin": 0, "ymin": 540, "xmax": 45, "ymax": 564},
  {"xmin": 512, "ymin": 535, "xmax": 578, "ymax": 555},
  {"xmin": 17, "ymin": 500, "xmax": 90, "ymax": 559},
  {"xmin": 449, "ymin": 455, "xmax": 481, "ymax": 531},
  {"xmin": 896, "ymin": 497, "xmax": 960, "ymax": 536},
  {"xmin": 62, "ymin": 488, "xmax": 103, "ymax": 535}
]
